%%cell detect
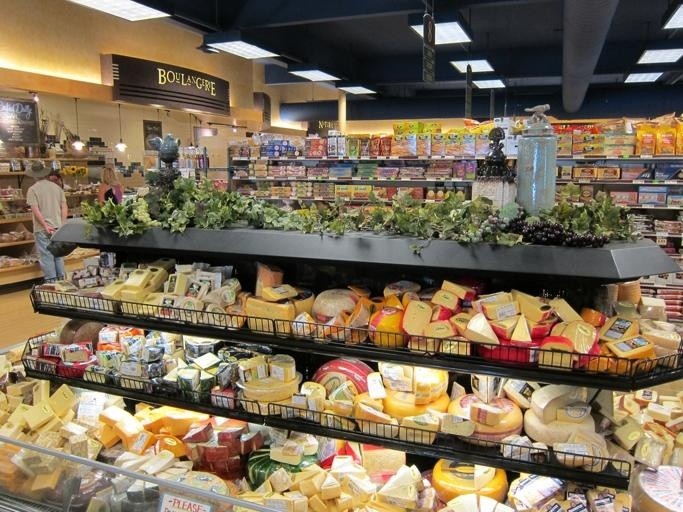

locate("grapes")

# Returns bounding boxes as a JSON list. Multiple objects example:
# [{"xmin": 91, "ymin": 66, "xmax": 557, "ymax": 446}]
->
[{"xmin": 469, "ymin": 206, "xmax": 611, "ymax": 248}]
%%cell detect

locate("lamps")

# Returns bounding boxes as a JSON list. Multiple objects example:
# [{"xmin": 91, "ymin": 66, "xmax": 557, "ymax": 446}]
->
[
  {"xmin": 70, "ymin": 0, "xmax": 176, "ymax": 22},
  {"xmin": 205, "ymin": 27, "xmax": 380, "ymax": 95},
  {"xmin": 71, "ymin": 97, "xmax": 127, "ymax": 152},
  {"xmin": 407, "ymin": 6, "xmax": 509, "ymax": 89},
  {"xmin": 624, "ymin": 1, "xmax": 683, "ymax": 83}
]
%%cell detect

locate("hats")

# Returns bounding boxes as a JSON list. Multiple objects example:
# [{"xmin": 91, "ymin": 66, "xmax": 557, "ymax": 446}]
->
[{"xmin": 24, "ymin": 160, "xmax": 52, "ymax": 178}]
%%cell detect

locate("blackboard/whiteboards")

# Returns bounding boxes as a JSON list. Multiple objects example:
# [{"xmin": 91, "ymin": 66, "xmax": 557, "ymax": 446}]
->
[{"xmin": 0, "ymin": 96, "xmax": 42, "ymax": 147}]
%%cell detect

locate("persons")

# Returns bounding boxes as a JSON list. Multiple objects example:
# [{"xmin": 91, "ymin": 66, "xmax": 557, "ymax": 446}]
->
[
  {"xmin": 23, "ymin": 161, "xmax": 69, "ymax": 284},
  {"xmin": 47, "ymin": 171, "xmax": 64, "ymax": 190},
  {"xmin": 95, "ymin": 166, "xmax": 122, "ymax": 205}
]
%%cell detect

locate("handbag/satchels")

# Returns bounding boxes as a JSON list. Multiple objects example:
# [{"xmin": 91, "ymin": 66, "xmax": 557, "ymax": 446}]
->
[{"xmin": 103, "ymin": 188, "xmax": 118, "ymax": 205}]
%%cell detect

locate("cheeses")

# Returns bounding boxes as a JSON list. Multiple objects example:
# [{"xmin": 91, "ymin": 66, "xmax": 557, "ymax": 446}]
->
[{"xmin": 1, "ymin": 257, "xmax": 683, "ymax": 511}]
